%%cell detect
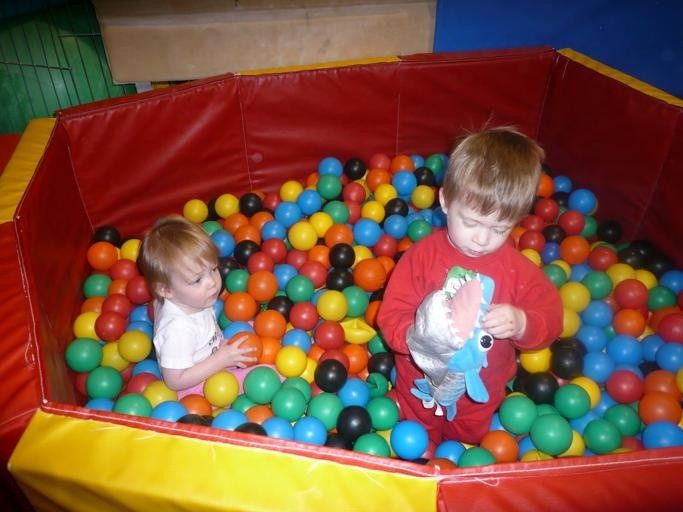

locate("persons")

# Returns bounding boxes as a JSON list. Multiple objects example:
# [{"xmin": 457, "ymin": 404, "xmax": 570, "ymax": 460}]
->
[
  {"xmin": 373, "ymin": 114, "xmax": 564, "ymax": 446},
  {"xmin": 135, "ymin": 215, "xmax": 286, "ymax": 410}
]
